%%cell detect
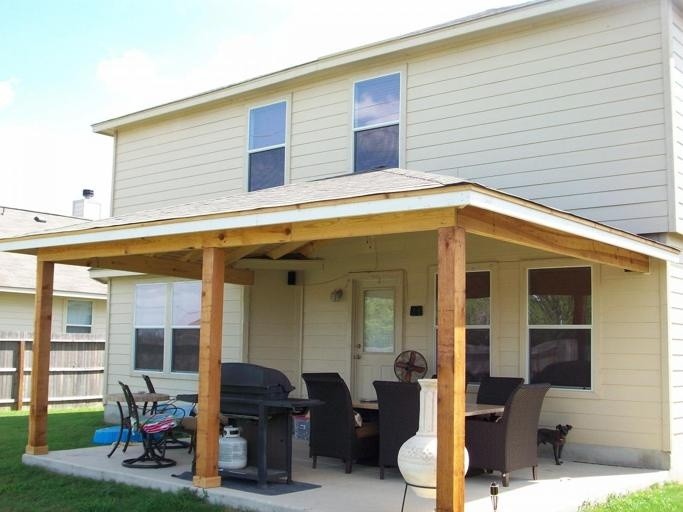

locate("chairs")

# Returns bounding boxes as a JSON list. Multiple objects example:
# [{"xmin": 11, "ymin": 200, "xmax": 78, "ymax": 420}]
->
[
  {"xmin": 119, "ymin": 380, "xmax": 185, "ymax": 469},
  {"xmin": 142, "ymin": 374, "xmax": 189, "ymax": 449},
  {"xmin": 301, "ymin": 372, "xmax": 551, "ymax": 487}
]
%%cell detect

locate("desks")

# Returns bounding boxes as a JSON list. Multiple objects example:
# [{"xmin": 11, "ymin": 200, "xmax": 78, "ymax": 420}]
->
[{"xmin": 106, "ymin": 393, "xmax": 169, "ymax": 458}]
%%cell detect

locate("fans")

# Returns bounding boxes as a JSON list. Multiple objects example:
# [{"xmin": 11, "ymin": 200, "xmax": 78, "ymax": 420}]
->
[{"xmin": 394, "ymin": 350, "xmax": 428, "ymax": 384}]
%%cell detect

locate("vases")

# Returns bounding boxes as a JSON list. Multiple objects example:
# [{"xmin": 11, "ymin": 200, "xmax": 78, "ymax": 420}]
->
[{"xmin": 397, "ymin": 379, "xmax": 470, "ymax": 499}]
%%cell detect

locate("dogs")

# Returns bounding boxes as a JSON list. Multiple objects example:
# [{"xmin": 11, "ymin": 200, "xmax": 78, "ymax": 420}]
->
[{"xmin": 537, "ymin": 423, "xmax": 572, "ymax": 465}]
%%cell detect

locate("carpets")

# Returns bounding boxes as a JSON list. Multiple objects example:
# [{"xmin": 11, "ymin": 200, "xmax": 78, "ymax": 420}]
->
[{"xmin": 171, "ymin": 471, "xmax": 322, "ymax": 495}]
